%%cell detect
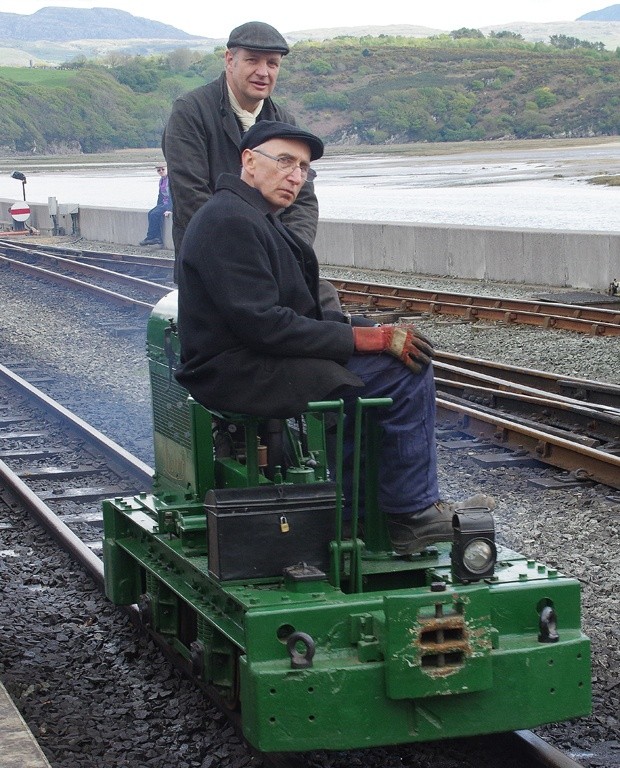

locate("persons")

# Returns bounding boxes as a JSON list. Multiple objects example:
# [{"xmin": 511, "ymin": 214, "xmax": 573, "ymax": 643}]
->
[
  {"xmin": 139, "ymin": 163, "xmax": 174, "ymax": 245},
  {"xmin": 173, "ymin": 121, "xmax": 497, "ymax": 554},
  {"xmin": 161, "ymin": 22, "xmax": 345, "ymax": 313}
]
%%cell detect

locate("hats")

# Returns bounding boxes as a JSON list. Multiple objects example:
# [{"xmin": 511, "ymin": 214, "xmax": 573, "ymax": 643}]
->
[
  {"xmin": 239, "ymin": 120, "xmax": 323, "ymax": 162},
  {"xmin": 155, "ymin": 163, "xmax": 166, "ymax": 168},
  {"xmin": 227, "ymin": 21, "xmax": 290, "ymax": 56}
]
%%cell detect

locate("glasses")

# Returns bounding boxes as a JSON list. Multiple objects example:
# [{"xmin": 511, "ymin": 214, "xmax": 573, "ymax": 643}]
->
[
  {"xmin": 157, "ymin": 168, "xmax": 165, "ymax": 172},
  {"xmin": 252, "ymin": 149, "xmax": 317, "ymax": 182}
]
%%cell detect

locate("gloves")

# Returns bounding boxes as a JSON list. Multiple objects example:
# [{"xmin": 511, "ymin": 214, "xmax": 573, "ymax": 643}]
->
[{"xmin": 353, "ymin": 325, "xmax": 436, "ymax": 372}]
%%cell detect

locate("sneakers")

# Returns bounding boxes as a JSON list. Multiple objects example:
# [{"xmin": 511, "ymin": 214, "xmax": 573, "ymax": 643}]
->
[{"xmin": 386, "ymin": 494, "xmax": 495, "ymax": 554}]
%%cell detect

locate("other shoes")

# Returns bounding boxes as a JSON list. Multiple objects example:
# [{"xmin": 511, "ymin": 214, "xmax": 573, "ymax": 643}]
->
[{"xmin": 139, "ymin": 237, "xmax": 162, "ymax": 245}]
